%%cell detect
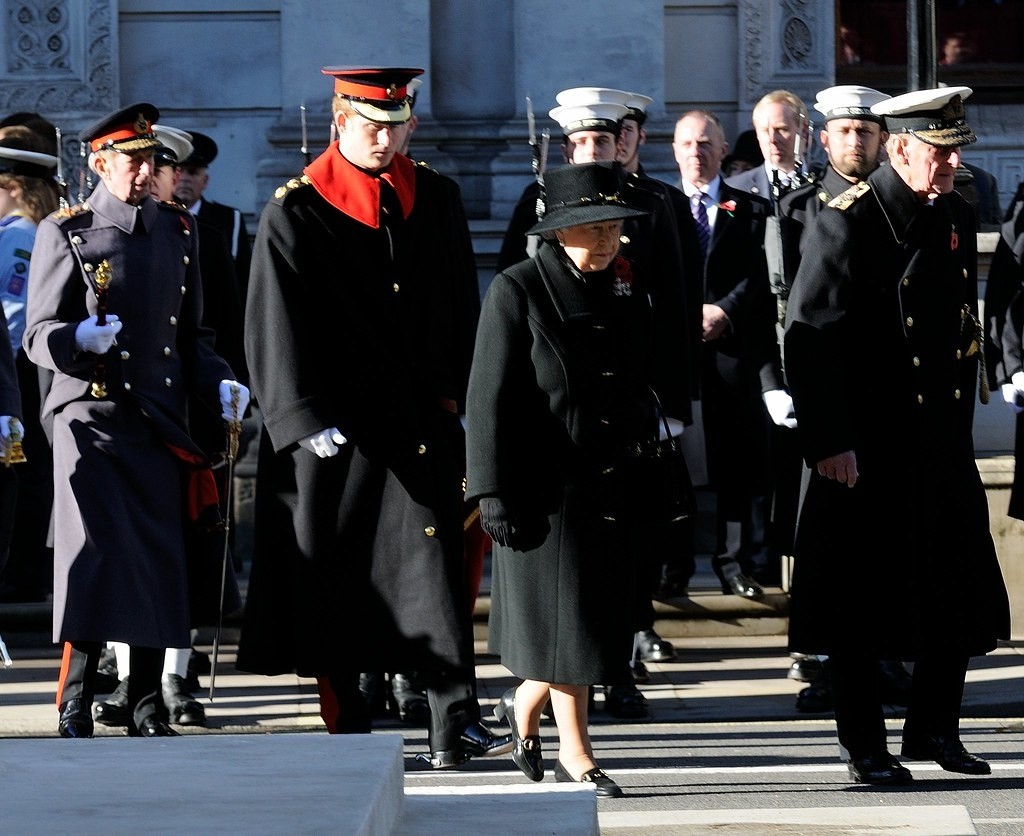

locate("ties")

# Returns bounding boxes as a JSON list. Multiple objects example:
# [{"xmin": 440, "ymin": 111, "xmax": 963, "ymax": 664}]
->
[{"xmin": 691, "ymin": 192, "xmax": 711, "ymax": 265}]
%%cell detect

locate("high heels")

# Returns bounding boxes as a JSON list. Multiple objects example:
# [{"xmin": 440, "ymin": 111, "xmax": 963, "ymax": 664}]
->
[
  {"xmin": 555, "ymin": 755, "xmax": 624, "ymax": 799},
  {"xmin": 493, "ymin": 686, "xmax": 546, "ymax": 783}
]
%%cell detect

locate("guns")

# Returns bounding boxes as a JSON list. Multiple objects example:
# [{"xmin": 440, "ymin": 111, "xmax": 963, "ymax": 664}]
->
[
  {"xmin": 56, "ymin": 125, "xmax": 95, "ymax": 208},
  {"xmin": 525, "ymin": 95, "xmax": 551, "ymax": 220},
  {"xmin": 770, "ymin": 106, "xmax": 812, "ymax": 383},
  {"xmin": 301, "ymin": 102, "xmax": 338, "ymax": 166}
]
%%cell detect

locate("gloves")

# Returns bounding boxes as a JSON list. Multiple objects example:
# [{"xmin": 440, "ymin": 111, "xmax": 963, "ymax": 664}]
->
[
  {"xmin": 478, "ymin": 495, "xmax": 525, "ymax": 554},
  {"xmin": 0, "ymin": 415, "xmax": 25, "ymax": 457},
  {"xmin": 298, "ymin": 427, "xmax": 347, "ymax": 458},
  {"xmin": 1012, "ymin": 371, "xmax": 1024, "ymax": 398},
  {"xmin": 762, "ymin": 390, "xmax": 798, "ymax": 428},
  {"xmin": 218, "ymin": 380, "xmax": 250, "ymax": 424},
  {"xmin": 659, "ymin": 417, "xmax": 685, "ymax": 442},
  {"xmin": 75, "ymin": 315, "xmax": 122, "ymax": 354},
  {"xmin": 1002, "ymin": 383, "xmax": 1018, "ymax": 405}
]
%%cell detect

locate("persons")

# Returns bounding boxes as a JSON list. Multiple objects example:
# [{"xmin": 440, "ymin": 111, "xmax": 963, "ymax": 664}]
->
[
  {"xmin": 782, "ymin": 86, "xmax": 1012, "ymax": 789},
  {"xmin": 937, "ymin": 38, "xmax": 976, "ymax": 67},
  {"xmin": 0, "ymin": 40, "xmax": 1024, "ymax": 755},
  {"xmin": 464, "ymin": 161, "xmax": 660, "ymax": 797},
  {"xmin": 839, "ymin": 26, "xmax": 874, "ymax": 85},
  {"xmin": 244, "ymin": 64, "xmax": 482, "ymax": 771},
  {"xmin": 22, "ymin": 101, "xmax": 251, "ymax": 740}
]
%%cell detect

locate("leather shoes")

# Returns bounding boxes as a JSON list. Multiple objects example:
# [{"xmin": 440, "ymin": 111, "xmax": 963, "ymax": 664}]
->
[
  {"xmin": 901, "ymin": 737, "xmax": 993, "ymax": 775},
  {"xmin": 797, "ymin": 685, "xmax": 834, "ymax": 713},
  {"xmin": 463, "ymin": 723, "xmax": 514, "ymax": 755},
  {"xmin": 787, "ymin": 660, "xmax": 823, "ymax": 680},
  {"xmin": 638, "ymin": 628, "xmax": 680, "ymax": 663},
  {"xmin": 630, "ymin": 667, "xmax": 650, "ymax": 684},
  {"xmin": 95, "ymin": 675, "xmax": 129, "ymax": 725},
  {"xmin": 847, "ymin": 754, "xmax": 914, "ymax": 789},
  {"xmin": 189, "ymin": 651, "xmax": 211, "ymax": 672},
  {"xmin": 162, "ymin": 674, "xmax": 207, "ymax": 724},
  {"xmin": 394, "ymin": 673, "xmax": 430, "ymax": 723},
  {"xmin": 140, "ymin": 715, "xmax": 183, "ymax": 737},
  {"xmin": 432, "ymin": 749, "xmax": 471, "ymax": 770},
  {"xmin": 600, "ymin": 687, "xmax": 652, "ymax": 721},
  {"xmin": 58, "ymin": 698, "xmax": 95, "ymax": 739},
  {"xmin": 361, "ymin": 672, "xmax": 403, "ymax": 728},
  {"xmin": 723, "ymin": 575, "xmax": 764, "ymax": 597}
]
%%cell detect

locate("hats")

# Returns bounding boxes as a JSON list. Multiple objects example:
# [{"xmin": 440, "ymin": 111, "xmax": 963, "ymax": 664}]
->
[
  {"xmin": 81, "ymin": 101, "xmax": 160, "ymax": 154},
  {"xmin": 182, "ymin": 130, "xmax": 219, "ymax": 167},
  {"xmin": 813, "ymin": 85, "xmax": 893, "ymax": 133},
  {"xmin": 549, "ymin": 85, "xmax": 656, "ymax": 137},
  {"xmin": 0, "ymin": 138, "xmax": 58, "ymax": 178},
  {"xmin": 318, "ymin": 64, "xmax": 424, "ymax": 126},
  {"xmin": 523, "ymin": 162, "xmax": 651, "ymax": 237},
  {"xmin": 152, "ymin": 124, "xmax": 195, "ymax": 164},
  {"xmin": 870, "ymin": 86, "xmax": 979, "ymax": 146}
]
